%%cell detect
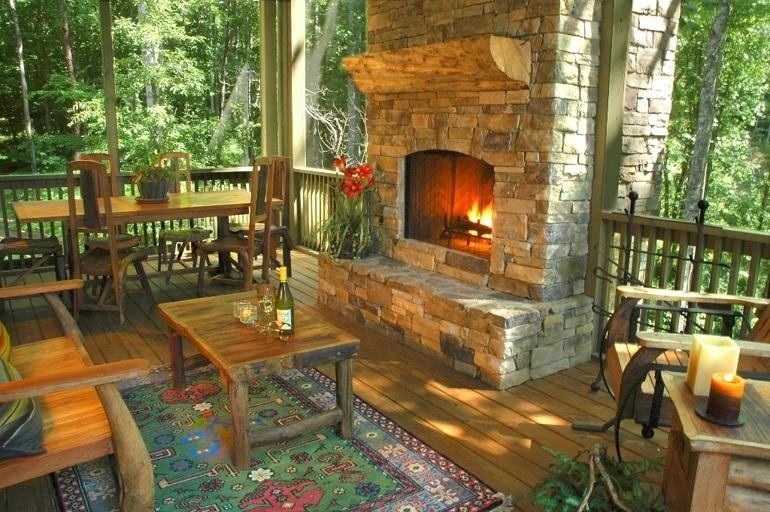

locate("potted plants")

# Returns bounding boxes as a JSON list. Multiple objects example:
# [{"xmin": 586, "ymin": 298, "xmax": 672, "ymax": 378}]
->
[{"xmin": 129, "ymin": 165, "xmax": 174, "ymax": 203}]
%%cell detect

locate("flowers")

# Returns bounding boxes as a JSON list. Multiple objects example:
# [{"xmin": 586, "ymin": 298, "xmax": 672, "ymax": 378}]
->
[{"xmin": 315, "ymin": 154, "xmax": 381, "ymax": 233}]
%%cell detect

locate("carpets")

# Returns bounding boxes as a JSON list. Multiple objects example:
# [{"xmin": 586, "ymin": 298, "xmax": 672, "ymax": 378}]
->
[{"xmin": 46, "ymin": 363, "xmax": 514, "ymax": 511}]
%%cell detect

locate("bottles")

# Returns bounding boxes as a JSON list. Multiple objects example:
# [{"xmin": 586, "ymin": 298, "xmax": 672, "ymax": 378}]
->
[{"xmin": 274, "ymin": 265, "xmax": 295, "ymax": 335}]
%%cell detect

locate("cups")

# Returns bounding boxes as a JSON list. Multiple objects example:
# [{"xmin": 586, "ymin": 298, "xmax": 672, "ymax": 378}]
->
[
  {"xmin": 239, "ymin": 306, "xmax": 256, "ymax": 329},
  {"xmin": 234, "ymin": 299, "xmax": 250, "ymax": 321},
  {"xmin": 255, "ymin": 283, "xmax": 276, "ymax": 327},
  {"xmin": 249, "ymin": 320, "xmax": 295, "ymax": 343}
]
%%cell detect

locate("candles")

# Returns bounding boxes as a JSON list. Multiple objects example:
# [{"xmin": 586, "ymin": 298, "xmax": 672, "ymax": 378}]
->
[{"xmin": 707, "ymin": 372, "xmax": 746, "ymax": 424}]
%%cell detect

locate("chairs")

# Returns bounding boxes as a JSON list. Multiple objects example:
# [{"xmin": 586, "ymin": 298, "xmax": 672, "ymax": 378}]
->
[
  {"xmin": 78, "ymin": 151, "xmax": 141, "ymax": 297},
  {"xmin": 194, "ymin": 157, "xmax": 274, "ymax": 298},
  {"xmin": 0, "ymin": 235, "xmax": 74, "ymax": 311},
  {"xmin": 230, "ymin": 156, "xmax": 291, "ymax": 280},
  {"xmin": 160, "ymin": 152, "xmax": 212, "ymax": 265},
  {"xmin": 66, "ymin": 160, "xmax": 156, "ymax": 324}
]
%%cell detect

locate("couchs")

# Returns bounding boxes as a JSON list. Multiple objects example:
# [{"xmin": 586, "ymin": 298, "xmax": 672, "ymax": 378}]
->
[
  {"xmin": 571, "ymin": 285, "xmax": 770, "ymax": 463},
  {"xmin": 1, "ymin": 277, "xmax": 155, "ymax": 511}
]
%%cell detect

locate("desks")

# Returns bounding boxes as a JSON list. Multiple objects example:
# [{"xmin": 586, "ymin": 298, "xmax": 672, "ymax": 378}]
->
[
  {"xmin": 158, "ymin": 289, "xmax": 360, "ymax": 470},
  {"xmin": 661, "ymin": 369, "xmax": 770, "ymax": 512}
]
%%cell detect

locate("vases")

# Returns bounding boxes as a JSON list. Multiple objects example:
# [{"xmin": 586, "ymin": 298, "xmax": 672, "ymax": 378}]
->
[{"xmin": 336, "ymin": 191, "xmax": 369, "ymax": 259}]
